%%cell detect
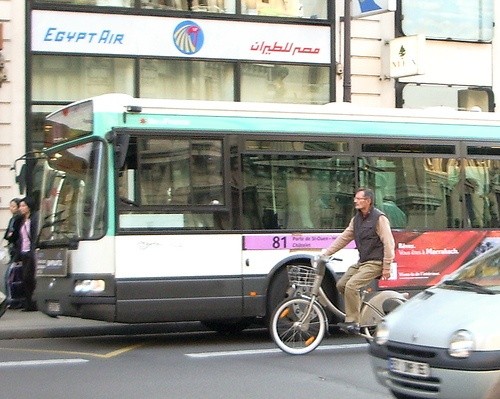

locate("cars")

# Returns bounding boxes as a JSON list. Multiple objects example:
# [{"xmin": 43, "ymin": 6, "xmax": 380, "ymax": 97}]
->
[{"xmin": 369, "ymin": 245, "xmax": 500, "ymax": 399}]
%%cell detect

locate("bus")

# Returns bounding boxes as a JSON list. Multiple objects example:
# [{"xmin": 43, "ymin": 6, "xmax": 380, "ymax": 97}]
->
[{"xmin": 11, "ymin": 93, "xmax": 500, "ymax": 335}]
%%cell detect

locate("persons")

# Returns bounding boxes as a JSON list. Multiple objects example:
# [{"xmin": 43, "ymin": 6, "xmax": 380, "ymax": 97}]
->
[
  {"xmin": 4, "ymin": 198, "xmax": 25, "ymax": 309},
  {"xmin": 381, "ymin": 194, "xmax": 408, "ymax": 229},
  {"xmin": 141, "ymin": 163, "xmax": 168, "ymax": 204},
  {"xmin": 18, "ymin": 197, "xmax": 38, "ymax": 312},
  {"xmin": 317, "ymin": 189, "xmax": 395, "ymax": 334},
  {"xmin": 465, "ymin": 178, "xmax": 485, "ymax": 227}
]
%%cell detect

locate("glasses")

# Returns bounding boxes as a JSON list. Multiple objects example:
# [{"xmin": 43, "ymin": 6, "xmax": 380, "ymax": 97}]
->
[{"xmin": 353, "ymin": 196, "xmax": 368, "ymax": 203}]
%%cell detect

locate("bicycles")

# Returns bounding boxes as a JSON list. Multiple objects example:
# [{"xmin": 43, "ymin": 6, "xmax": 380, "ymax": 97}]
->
[{"xmin": 269, "ymin": 253, "xmax": 409, "ymax": 356}]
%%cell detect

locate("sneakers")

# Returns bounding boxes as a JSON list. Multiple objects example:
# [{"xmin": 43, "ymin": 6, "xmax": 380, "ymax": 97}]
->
[{"xmin": 338, "ymin": 322, "xmax": 360, "ymax": 332}]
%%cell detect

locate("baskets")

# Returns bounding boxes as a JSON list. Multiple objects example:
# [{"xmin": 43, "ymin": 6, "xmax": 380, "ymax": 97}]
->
[{"xmin": 287, "ymin": 265, "xmax": 316, "ymax": 287}]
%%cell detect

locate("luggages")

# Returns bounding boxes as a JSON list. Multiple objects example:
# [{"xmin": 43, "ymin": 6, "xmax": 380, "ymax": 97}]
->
[{"xmin": 7, "ymin": 260, "xmax": 26, "ymax": 309}]
